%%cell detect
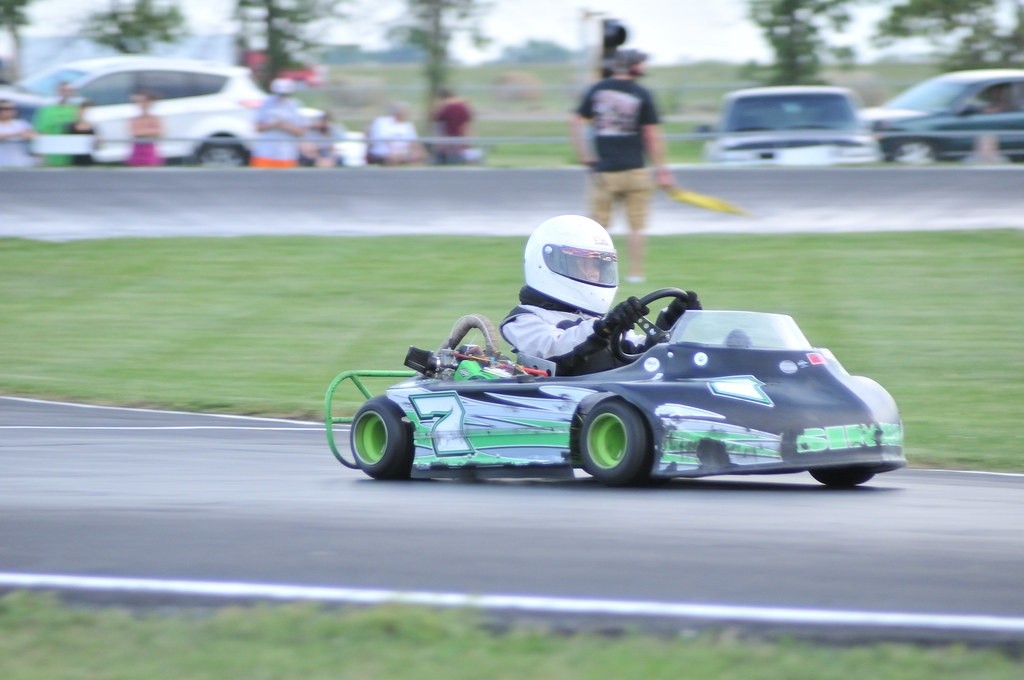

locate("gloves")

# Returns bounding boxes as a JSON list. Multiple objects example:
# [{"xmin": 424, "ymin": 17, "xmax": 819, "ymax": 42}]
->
[
  {"xmin": 593, "ymin": 297, "xmax": 649, "ymax": 338},
  {"xmin": 667, "ymin": 290, "xmax": 701, "ymax": 325}
]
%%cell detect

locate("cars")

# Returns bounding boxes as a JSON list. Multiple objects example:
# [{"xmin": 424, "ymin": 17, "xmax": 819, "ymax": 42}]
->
[
  {"xmin": 692, "ymin": 83, "xmax": 888, "ymax": 165},
  {"xmin": 862, "ymin": 67, "xmax": 1024, "ymax": 161},
  {"xmin": 0, "ymin": 55, "xmax": 263, "ymax": 169}
]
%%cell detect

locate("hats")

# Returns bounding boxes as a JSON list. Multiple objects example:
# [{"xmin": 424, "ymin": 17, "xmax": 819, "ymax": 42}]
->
[{"xmin": 271, "ymin": 78, "xmax": 294, "ymax": 95}]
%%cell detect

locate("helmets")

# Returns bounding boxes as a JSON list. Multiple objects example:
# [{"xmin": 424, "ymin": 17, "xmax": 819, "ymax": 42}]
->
[{"xmin": 524, "ymin": 214, "xmax": 618, "ymax": 314}]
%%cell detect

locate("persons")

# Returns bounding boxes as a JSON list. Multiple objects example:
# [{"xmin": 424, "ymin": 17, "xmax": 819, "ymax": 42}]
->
[
  {"xmin": 966, "ymin": 83, "xmax": 1018, "ymax": 114},
  {"xmin": 367, "ymin": 102, "xmax": 424, "ymax": 166},
  {"xmin": 126, "ymin": 92, "xmax": 163, "ymax": 166},
  {"xmin": 963, "ymin": 128, "xmax": 1011, "ymax": 163},
  {"xmin": 571, "ymin": 50, "xmax": 676, "ymax": 281},
  {"xmin": 0, "ymin": 97, "xmax": 39, "ymax": 166},
  {"xmin": 500, "ymin": 215, "xmax": 752, "ymax": 377},
  {"xmin": 302, "ymin": 112, "xmax": 343, "ymax": 166},
  {"xmin": 431, "ymin": 87, "xmax": 475, "ymax": 156},
  {"xmin": 70, "ymin": 102, "xmax": 100, "ymax": 166},
  {"xmin": 28, "ymin": 82, "xmax": 78, "ymax": 167},
  {"xmin": 249, "ymin": 79, "xmax": 309, "ymax": 166}
]
